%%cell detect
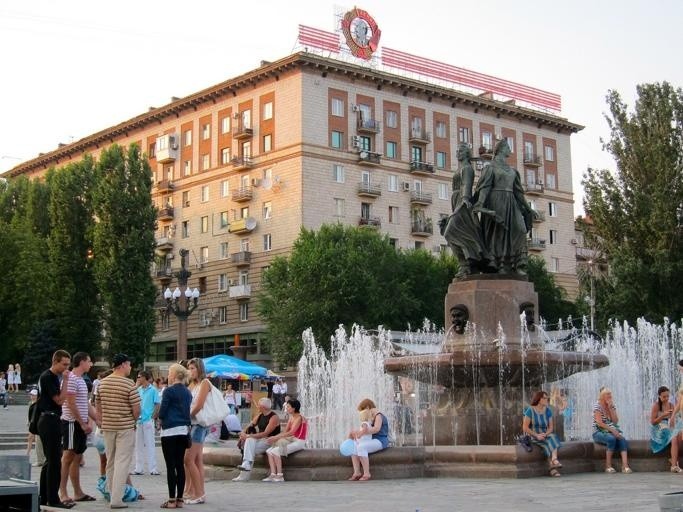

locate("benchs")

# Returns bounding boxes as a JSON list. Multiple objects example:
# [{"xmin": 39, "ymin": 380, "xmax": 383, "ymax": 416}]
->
[{"xmin": 0, "ymin": 478, "xmax": 40, "ymax": 512}]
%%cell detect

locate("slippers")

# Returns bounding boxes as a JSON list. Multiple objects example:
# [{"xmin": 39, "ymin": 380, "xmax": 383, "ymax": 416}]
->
[
  {"xmin": 160, "ymin": 499, "xmax": 177, "ymax": 509},
  {"xmin": 61, "ymin": 499, "xmax": 77, "ymax": 508},
  {"xmin": 73, "ymin": 494, "xmax": 96, "ymax": 502},
  {"xmin": 176, "ymin": 499, "xmax": 185, "ymax": 509}
]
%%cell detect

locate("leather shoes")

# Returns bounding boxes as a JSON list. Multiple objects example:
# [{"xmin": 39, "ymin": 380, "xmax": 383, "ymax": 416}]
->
[{"xmin": 49, "ymin": 502, "xmax": 70, "ymax": 509}]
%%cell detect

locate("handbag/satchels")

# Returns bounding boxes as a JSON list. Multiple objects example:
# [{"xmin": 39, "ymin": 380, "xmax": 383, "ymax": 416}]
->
[
  {"xmin": 185, "ymin": 430, "xmax": 192, "ymax": 450},
  {"xmin": 517, "ymin": 433, "xmax": 533, "ymax": 453},
  {"xmin": 96, "ymin": 476, "xmax": 140, "ymax": 503},
  {"xmin": 193, "ymin": 386, "xmax": 232, "ymax": 430},
  {"xmin": 272, "ymin": 436, "xmax": 295, "ymax": 448}
]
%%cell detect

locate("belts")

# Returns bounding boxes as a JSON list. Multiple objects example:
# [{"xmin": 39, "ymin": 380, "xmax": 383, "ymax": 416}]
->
[{"xmin": 40, "ymin": 411, "xmax": 60, "ymax": 417}]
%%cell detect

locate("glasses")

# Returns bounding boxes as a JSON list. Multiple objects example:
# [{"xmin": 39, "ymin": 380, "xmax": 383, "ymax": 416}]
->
[{"xmin": 136, "ymin": 376, "xmax": 141, "ymax": 379}]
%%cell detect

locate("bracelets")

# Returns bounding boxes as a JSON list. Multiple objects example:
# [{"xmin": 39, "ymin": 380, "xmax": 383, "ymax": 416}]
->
[{"xmin": 247, "ymin": 434, "xmax": 251, "ymax": 437}]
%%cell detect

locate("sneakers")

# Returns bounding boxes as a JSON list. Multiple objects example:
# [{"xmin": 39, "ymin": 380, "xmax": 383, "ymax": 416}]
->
[
  {"xmin": 184, "ymin": 494, "xmax": 206, "ymax": 505},
  {"xmin": 670, "ymin": 466, "xmax": 683, "ymax": 474},
  {"xmin": 604, "ymin": 468, "xmax": 617, "ymax": 474},
  {"xmin": 110, "ymin": 502, "xmax": 129, "ymax": 510},
  {"xmin": 236, "ymin": 464, "xmax": 251, "ymax": 472},
  {"xmin": 31, "ymin": 462, "xmax": 43, "ymax": 467},
  {"xmin": 231, "ymin": 475, "xmax": 249, "ymax": 482},
  {"xmin": 622, "ymin": 466, "xmax": 633, "ymax": 474}
]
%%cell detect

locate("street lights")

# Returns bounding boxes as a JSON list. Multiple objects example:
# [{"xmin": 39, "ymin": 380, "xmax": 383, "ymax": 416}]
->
[{"xmin": 164, "ymin": 288, "xmax": 199, "ymax": 365}]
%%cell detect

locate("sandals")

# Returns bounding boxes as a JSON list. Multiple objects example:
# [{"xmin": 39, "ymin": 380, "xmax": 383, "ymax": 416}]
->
[
  {"xmin": 261, "ymin": 477, "xmax": 272, "ymax": 482},
  {"xmin": 549, "ymin": 470, "xmax": 561, "ymax": 478},
  {"xmin": 358, "ymin": 474, "xmax": 372, "ymax": 482},
  {"xmin": 348, "ymin": 474, "xmax": 361, "ymax": 480},
  {"xmin": 549, "ymin": 458, "xmax": 563, "ymax": 470},
  {"xmin": 271, "ymin": 477, "xmax": 285, "ymax": 483},
  {"xmin": 150, "ymin": 470, "xmax": 161, "ymax": 476},
  {"xmin": 129, "ymin": 470, "xmax": 145, "ymax": 476}
]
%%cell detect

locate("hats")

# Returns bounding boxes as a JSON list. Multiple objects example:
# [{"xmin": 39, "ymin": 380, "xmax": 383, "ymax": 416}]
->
[
  {"xmin": 111, "ymin": 353, "xmax": 137, "ymax": 369},
  {"xmin": 30, "ymin": 389, "xmax": 39, "ymax": 396}
]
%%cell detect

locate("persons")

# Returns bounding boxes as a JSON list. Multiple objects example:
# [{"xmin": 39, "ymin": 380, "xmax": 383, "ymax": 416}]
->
[
  {"xmin": 28, "ymin": 351, "xmax": 96, "ymax": 508},
  {"xmin": 355, "ymin": 409, "xmax": 372, "ymax": 442},
  {"xmin": 14, "ymin": 364, "xmax": 21, "ymax": 393},
  {"xmin": 88, "ymin": 353, "xmax": 141, "ymax": 507},
  {"xmin": 208, "ymin": 384, "xmax": 252, "ymax": 440},
  {"xmin": 348, "ymin": 399, "xmax": 389, "ymax": 480},
  {"xmin": 0, "ymin": 372, "xmax": 7, "ymax": 407},
  {"xmin": 154, "ymin": 357, "xmax": 213, "ymax": 508},
  {"xmin": 522, "ymin": 392, "xmax": 562, "ymax": 477},
  {"xmin": 476, "ymin": 138, "xmax": 539, "ymax": 275},
  {"xmin": 650, "ymin": 387, "xmax": 683, "ymax": 473},
  {"xmin": 592, "ymin": 389, "xmax": 633, "ymax": 474},
  {"xmin": 129, "ymin": 371, "xmax": 169, "ymax": 475},
  {"xmin": 670, "ymin": 360, "xmax": 683, "ymax": 438},
  {"xmin": 232, "ymin": 398, "xmax": 307, "ymax": 483},
  {"xmin": 6, "ymin": 363, "xmax": 14, "ymax": 392},
  {"xmin": 444, "ymin": 142, "xmax": 487, "ymax": 279},
  {"xmin": 260, "ymin": 378, "xmax": 289, "ymax": 411},
  {"xmin": 450, "ymin": 304, "xmax": 468, "ymax": 334},
  {"xmin": 520, "ymin": 302, "xmax": 534, "ymax": 331}
]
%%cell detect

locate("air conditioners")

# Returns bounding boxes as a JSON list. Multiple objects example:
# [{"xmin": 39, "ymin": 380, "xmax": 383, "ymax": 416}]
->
[
  {"xmin": 251, "ymin": 178, "xmax": 257, "ymax": 186},
  {"xmin": 353, "ymin": 141, "xmax": 360, "ymax": 146},
  {"xmin": 353, "ymin": 135, "xmax": 359, "ymax": 140},
  {"xmin": 476, "ymin": 164, "xmax": 483, "ymax": 169},
  {"xmin": 536, "ymin": 179, "xmax": 542, "ymax": 183},
  {"xmin": 402, "ymin": 181, "xmax": 410, "ymax": 191},
  {"xmin": 192, "ymin": 263, "xmax": 201, "ymax": 268},
  {"xmin": 353, "ymin": 105, "xmax": 359, "ymax": 111}
]
